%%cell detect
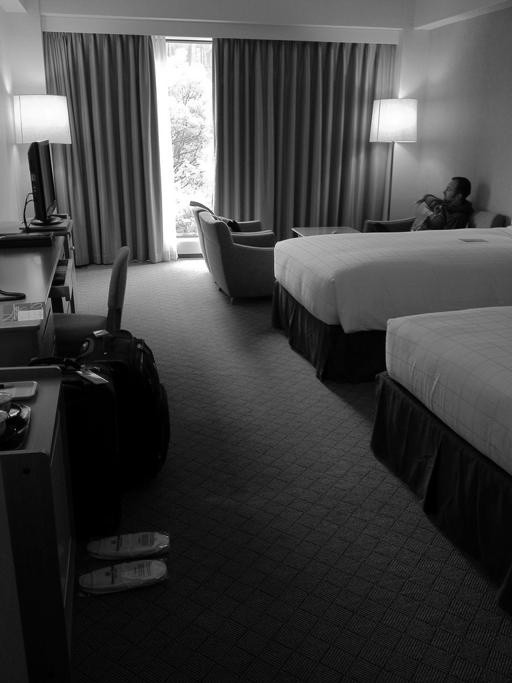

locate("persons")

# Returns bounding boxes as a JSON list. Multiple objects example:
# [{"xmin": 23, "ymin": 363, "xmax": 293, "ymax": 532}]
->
[{"xmin": 410, "ymin": 176, "xmax": 474, "ymax": 231}]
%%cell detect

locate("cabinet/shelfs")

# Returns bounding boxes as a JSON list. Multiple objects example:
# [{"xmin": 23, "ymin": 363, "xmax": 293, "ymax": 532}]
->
[{"xmin": 0, "ymin": 366, "xmax": 79, "ymax": 683}]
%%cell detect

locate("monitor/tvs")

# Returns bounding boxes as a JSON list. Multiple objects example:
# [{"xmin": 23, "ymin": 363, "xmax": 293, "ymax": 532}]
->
[{"xmin": 27, "ymin": 140, "xmax": 63, "ymax": 226}]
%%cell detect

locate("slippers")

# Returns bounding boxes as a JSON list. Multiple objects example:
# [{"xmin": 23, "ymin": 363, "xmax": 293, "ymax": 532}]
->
[{"xmin": 78, "ymin": 530, "xmax": 172, "ymax": 596}]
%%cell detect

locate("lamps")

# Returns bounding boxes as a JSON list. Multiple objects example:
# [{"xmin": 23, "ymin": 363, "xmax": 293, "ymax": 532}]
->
[
  {"xmin": 369, "ymin": 98, "xmax": 418, "ymax": 220},
  {"xmin": 13, "ymin": 94, "xmax": 73, "ymax": 146}
]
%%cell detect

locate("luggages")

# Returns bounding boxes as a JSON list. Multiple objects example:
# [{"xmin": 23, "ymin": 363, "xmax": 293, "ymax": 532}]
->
[
  {"xmin": 25, "ymin": 356, "xmax": 120, "ymax": 516},
  {"xmin": 81, "ymin": 330, "xmax": 174, "ymax": 486}
]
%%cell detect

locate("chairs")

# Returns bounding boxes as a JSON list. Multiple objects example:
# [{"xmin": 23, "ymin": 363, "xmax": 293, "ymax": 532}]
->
[
  {"xmin": 188, "ymin": 201, "xmax": 276, "ymax": 307},
  {"xmin": 363, "ymin": 209, "xmax": 507, "ymax": 230},
  {"xmin": 54, "ymin": 246, "xmax": 130, "ymax": 357}
]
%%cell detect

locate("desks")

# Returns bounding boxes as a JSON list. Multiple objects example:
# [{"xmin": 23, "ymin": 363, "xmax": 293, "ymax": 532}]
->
[{"xmin": 0, "ymin": 220, "xmax": 76, "ymax": 366}]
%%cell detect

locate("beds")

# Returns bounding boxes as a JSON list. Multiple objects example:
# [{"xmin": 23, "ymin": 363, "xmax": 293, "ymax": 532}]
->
[
  {"xmin": 274, "ymin": 225, "xmax": 511, "ymax": 384},
  {"xmin": 376, "ymin": 306, "xmax": 511, "ymax": 550}
]
